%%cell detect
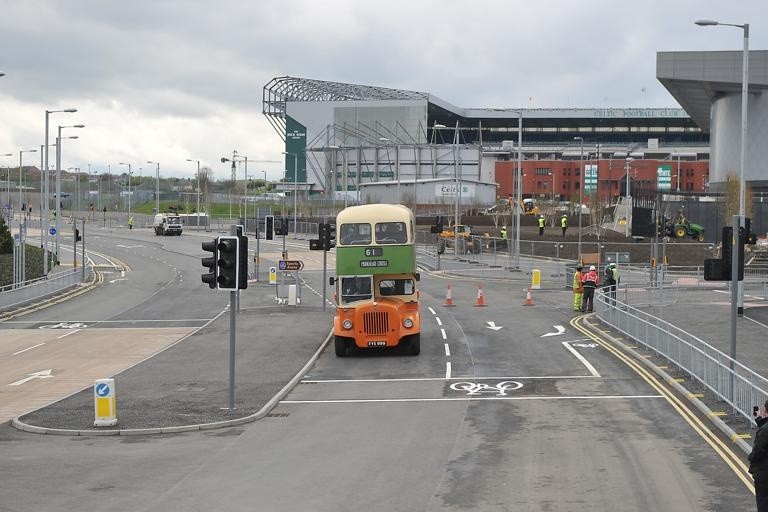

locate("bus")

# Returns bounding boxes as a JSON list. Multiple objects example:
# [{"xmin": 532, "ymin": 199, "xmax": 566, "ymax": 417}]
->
[{"xmin": 334, "ymin": 203, "xmax": 421, "ymax": 357}]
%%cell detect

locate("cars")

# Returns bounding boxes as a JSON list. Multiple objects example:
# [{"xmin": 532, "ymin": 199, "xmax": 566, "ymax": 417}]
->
[{"xmin": 462, "ymin": 197, "xmax": 588, "ymax": 216}]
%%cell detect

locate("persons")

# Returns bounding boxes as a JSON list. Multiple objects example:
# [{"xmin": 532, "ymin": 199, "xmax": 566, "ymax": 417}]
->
[
  {"xmin": 746, "ymin": 399, "xmax": 767, "ymax": 512},
  {"xmin": 571, "ymin": 261, "xmax": 620, "ymax": 312},
  {"xmin": 500, "ymin": 224, "xmax": 507, "ymax": 248},
  {"xmin": 559, "ymin": 214, "xmax": 568, "ymax": 238},
  {"xmin": 127, "ymin": 216, "xmax": 134, "ymax": 229},
  {"xmin": 537, "ymin": 214, "xmax": 545, "ymax": 236}
]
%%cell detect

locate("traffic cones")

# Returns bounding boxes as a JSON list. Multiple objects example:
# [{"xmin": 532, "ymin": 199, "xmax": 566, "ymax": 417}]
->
[
  {"xmin": 473, "ymin": 283, "xmax": 488, "ymax": 307},
  {"xmin": 522, "ymin": 287, "xmax": 535, "ymax": 306},
  {"xmin": 442, "ymin": 282, "xmax": 456, "ymax": 307}
]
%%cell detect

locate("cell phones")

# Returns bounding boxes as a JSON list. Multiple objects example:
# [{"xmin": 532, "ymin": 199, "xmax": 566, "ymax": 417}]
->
[{"xmin": 753, "ymin": 407, "xmax": 759, "ymax": 415}]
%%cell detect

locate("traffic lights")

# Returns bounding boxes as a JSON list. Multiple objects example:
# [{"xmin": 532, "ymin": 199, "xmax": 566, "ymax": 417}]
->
[
  {"xmin": 218, "ymin": 241, "xmax": 233, "ymax": 286},
  {"xmin": 200, "ymin": 240, "xmax": 217, "ymax": 290},
  {"xmin": 329, "ymin": 226, "xmax": 337, "ymax": 248}
]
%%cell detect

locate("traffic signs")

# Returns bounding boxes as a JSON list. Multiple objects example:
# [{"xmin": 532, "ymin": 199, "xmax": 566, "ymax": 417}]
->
[{"xmin": 280, "ymin": 261, "xmax": 303, "ymax": 270}]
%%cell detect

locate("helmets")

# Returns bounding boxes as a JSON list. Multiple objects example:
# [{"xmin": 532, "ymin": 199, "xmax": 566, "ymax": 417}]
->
[{"xmin": 590, "ymin": 266, "xmax": 595, "ymax": 270}]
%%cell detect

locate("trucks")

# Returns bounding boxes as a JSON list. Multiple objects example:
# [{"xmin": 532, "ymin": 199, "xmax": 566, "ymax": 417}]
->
[{"xmin": 605, "ymin": 195, "xmax": 624, "ymax": 209}]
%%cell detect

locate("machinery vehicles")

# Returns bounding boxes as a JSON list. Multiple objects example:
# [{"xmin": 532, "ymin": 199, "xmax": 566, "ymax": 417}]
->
[
  {"xmin": 657, "ymin": 215, "xmax": 706, "ymax": 241},
  {"xmin": 438, "ymin": 225, "xmax": 489, "ymax": 254}
]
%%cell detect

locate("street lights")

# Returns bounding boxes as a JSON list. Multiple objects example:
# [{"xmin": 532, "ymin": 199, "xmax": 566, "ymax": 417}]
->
[
  {"xmin": 677, "ymin": 154, "xmax": 681, "ymax": 193},
  {"xmin": 625, "ymin": 157, "xmax": 631, "ymax": 239},
  {"xmin": 86, "ymin": 146, "xmax": 359, "ymax": 240},
  {"xmin": 514, "ymin": 110, "xmax": 523, "ymax": 268},
  {"xmin": 695, "ymin": 18, "xmax": 750, "ymax": 403},
  {"xmin": 573, "ymin": 136, "xmax": 584, "ymax": 264},
  {"xmin": 1, "ymin": 109, "xmax": 85, "ymax": 273},
  {"xmin": 0, "ymin": 72, "xmax": 4, "ymax": 78},
  {"xmin": 379, "ymin": 137, "xmax": 400, "ymax": 204},
  {"xmin": 434, "ymin": 124, "xmax": 459, "ymax": 255},
  {"xmin": 524, "ymin": 173, "xmax": 535, "ymax": 199},
  {"xmin": 548, "ymin": 172, "xmax": 555, "ymax": 207}
]
